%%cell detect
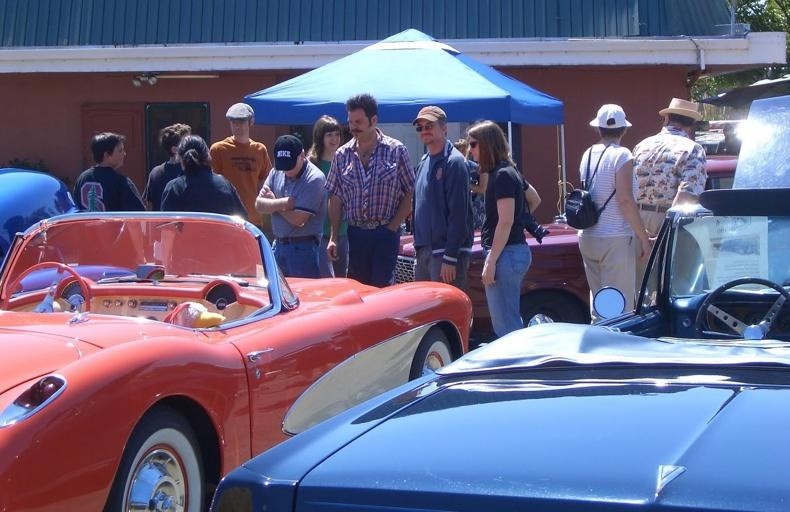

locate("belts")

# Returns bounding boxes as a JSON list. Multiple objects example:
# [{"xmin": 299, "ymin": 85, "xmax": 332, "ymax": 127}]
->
[
  {"xmin": 349, "ymin": 220, "xmax": 390, "ymax": 227},
  {"xmin": 273, "ymin": 236, "xmax": 313, "ymax": 243},
  {"xmin": 638, "ymin": 203, "xmax": 668, "ymax": 212}
]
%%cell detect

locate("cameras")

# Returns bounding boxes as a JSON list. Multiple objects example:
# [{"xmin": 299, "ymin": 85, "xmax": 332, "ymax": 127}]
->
[{"xmin": 524, "ymin": 212, "xmax": 550, "ymax": 244}]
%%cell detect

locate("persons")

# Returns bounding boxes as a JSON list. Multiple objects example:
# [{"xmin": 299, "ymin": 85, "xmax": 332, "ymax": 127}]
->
[
  {"xmin": 139, "ymin": 122, "xmax": 247, "ymax": 220},
  {"xmin": 210, "ymin": 103, "xmax": 272, "ymax": 228},
  {"xmin": 404, "ymin": 106, "xmax": 541, "ymax": 336},
  {"xmin": 715, "ymin": 122, "xmax": 741, "ymax": 155},
  {"xmin": 256, "ymin": 135, "xmax": 326, "ymax": 278},
  {"xmin": 306, "ymin": 114, "xmax": 348, "ymax": 278},
  {"xmin": 325, "ymin": 94, "xmax": 414, "ymax": 287},
  {"xmin": 71, "ymin": 132, "xmax": 146, "ymax": 214},
  {"xmin": 576, "ymin": 103, "xmax": 652, "ymax": 326},
  {"xmin": 631, "ymin": 96, "xmax": 710, "ymax": 310}
]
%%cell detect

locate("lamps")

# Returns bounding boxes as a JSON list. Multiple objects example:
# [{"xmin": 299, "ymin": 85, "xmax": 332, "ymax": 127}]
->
[{"xmin": 133, "ymin": 72, "xmax": 158, "ymax": 90}]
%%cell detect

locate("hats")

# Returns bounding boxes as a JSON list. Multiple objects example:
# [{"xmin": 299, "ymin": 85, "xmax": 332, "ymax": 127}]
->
[
  {"xmin": 273, "ymin": 134, "xmax": 303, "ymax": 170},
  {"xmin": 589, "ymin": 103, "xmax": 633, "ymax": 129},
  {"xmin": 658, "ymin": 97, "xmax": 703, "ymax": 122},
  {"xmin": 226, "ymin": 102, "xmax": 255, "ymax": 120},
  {"xmin": 414, "ymin": 106, "xmax": 446, "ymax": 126}
]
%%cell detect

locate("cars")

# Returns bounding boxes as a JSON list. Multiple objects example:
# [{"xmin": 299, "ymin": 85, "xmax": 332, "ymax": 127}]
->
[
  {"xmin": 694, "ymin": 120, "xmax": 746, "ymax": 154},
  {"xmin": 0, "ymin": 166, "xmax": 83, "ymax": 294},
  {"xmin": 391, "ymin": 154, "xmax": 741, "ymax": 346}
]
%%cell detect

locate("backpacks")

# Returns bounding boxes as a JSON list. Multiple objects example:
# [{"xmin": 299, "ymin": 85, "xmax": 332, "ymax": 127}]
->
[{"xmin": 565, "ymin": 143, "xmax": 616, "ymax": 228}]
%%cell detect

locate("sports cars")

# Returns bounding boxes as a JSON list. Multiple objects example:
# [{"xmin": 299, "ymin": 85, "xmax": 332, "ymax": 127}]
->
[
  {"xmin": 207, "ymin": 187, "xmax": 790, "ymax": 510},
  {"xmin": 1, "ymin": 210, "xmax": 474, "ymax": 512}
]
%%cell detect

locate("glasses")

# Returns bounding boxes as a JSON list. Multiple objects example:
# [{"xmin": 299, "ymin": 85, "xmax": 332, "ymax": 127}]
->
[
  {"xmin": 469, "ymin": 140, "xmax": 479, "ymax": 147},
  {"xmin": 416, "ymin": 124, "xmax": 433, "ymax": 132}
]
